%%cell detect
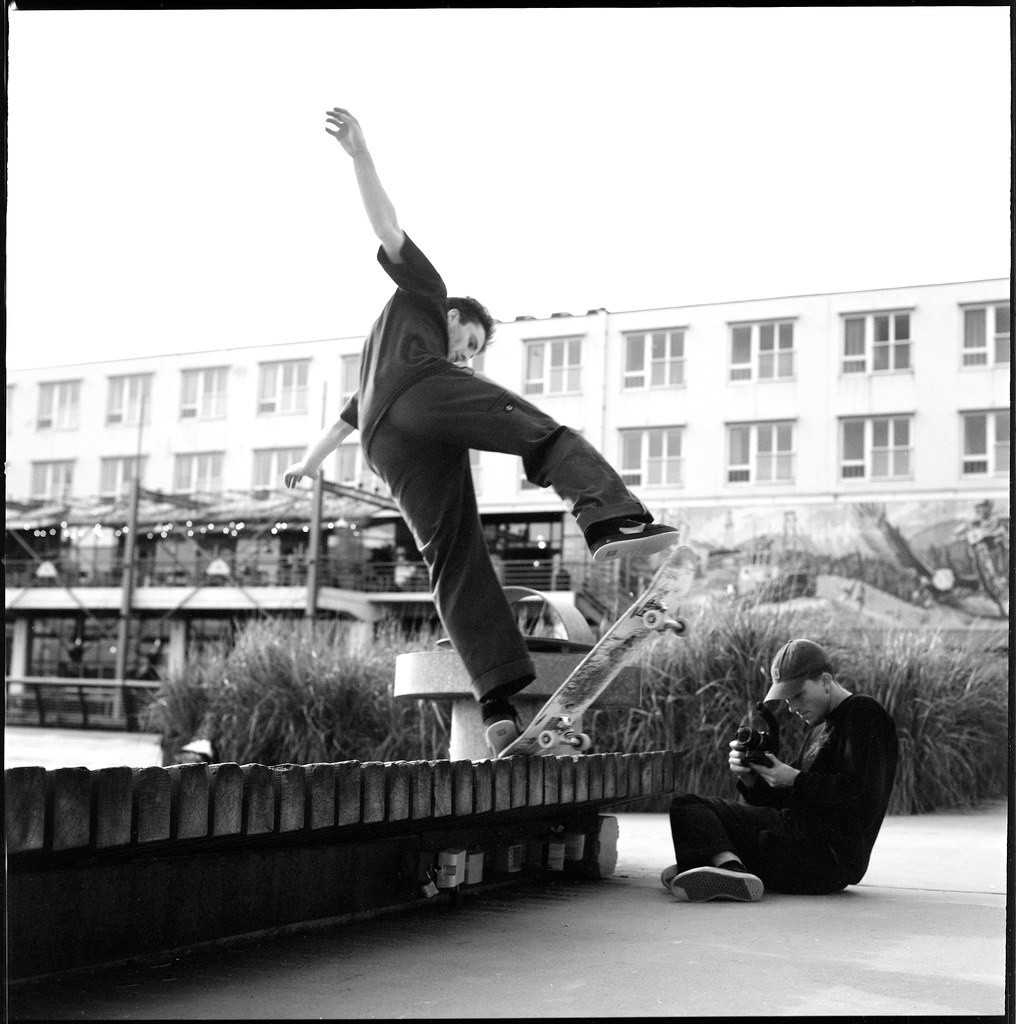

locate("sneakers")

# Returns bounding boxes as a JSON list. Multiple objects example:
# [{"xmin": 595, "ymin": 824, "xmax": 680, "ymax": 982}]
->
[
  {"xmin": 670, "ymin": 866, "xmax": 765, "ymax": 903},
  {"xmin": 479, "ymin": 694, "xmax": 525, "ymax": 760},
  {"xmin": 660, "ymin": 864, "xmax": 679, "ymax": 891},
  {"xmin": 584, "ymin": 517, "xmax": 681, "ymax": 562}
]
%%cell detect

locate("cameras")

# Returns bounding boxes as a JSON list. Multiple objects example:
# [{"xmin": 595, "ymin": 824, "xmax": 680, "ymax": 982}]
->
[{"xmin": 735, "ymin": 703, "xmax": 779, "ymax": 779}]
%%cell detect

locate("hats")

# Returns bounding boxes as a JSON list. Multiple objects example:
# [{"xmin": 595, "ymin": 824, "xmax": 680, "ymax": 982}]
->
[{"xmin": 763, "ymin": 639, "xmax": 832, "ymax": 704}]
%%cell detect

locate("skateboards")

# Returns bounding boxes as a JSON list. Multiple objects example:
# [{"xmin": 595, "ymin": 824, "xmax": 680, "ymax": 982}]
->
[{"xmin": 494, "ymin": 542, "xmax": 702, "ymax": 758}]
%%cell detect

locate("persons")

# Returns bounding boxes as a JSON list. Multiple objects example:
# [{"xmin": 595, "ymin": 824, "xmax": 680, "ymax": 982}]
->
[
  {"xmin": 660, "ymin": 638, "xmax": 900, "ymax": 904},
  {"xmin": 285, "ymin": 107, "xmax": 683, "ymax": 759}
]
